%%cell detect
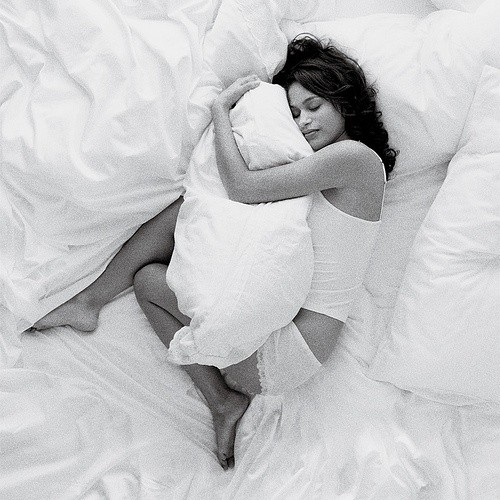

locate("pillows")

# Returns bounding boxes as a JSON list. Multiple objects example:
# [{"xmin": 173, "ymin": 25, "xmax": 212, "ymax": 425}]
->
[
  {"xmin": 367, "ymin": 65, "xmax": 500, "ymax": 408},
  {"xmin": 166, "ymin": 80, "xmax": 316, "ymax": 369},
  {"xmin": 279, "ymin": 10, "xmax": 486, "ymax": 183}
]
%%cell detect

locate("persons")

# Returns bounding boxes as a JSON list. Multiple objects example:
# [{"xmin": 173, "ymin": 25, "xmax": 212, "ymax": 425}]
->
[{"xmin": 31, "ymin": 32, "xmax": 401, "ymax": 471}]
